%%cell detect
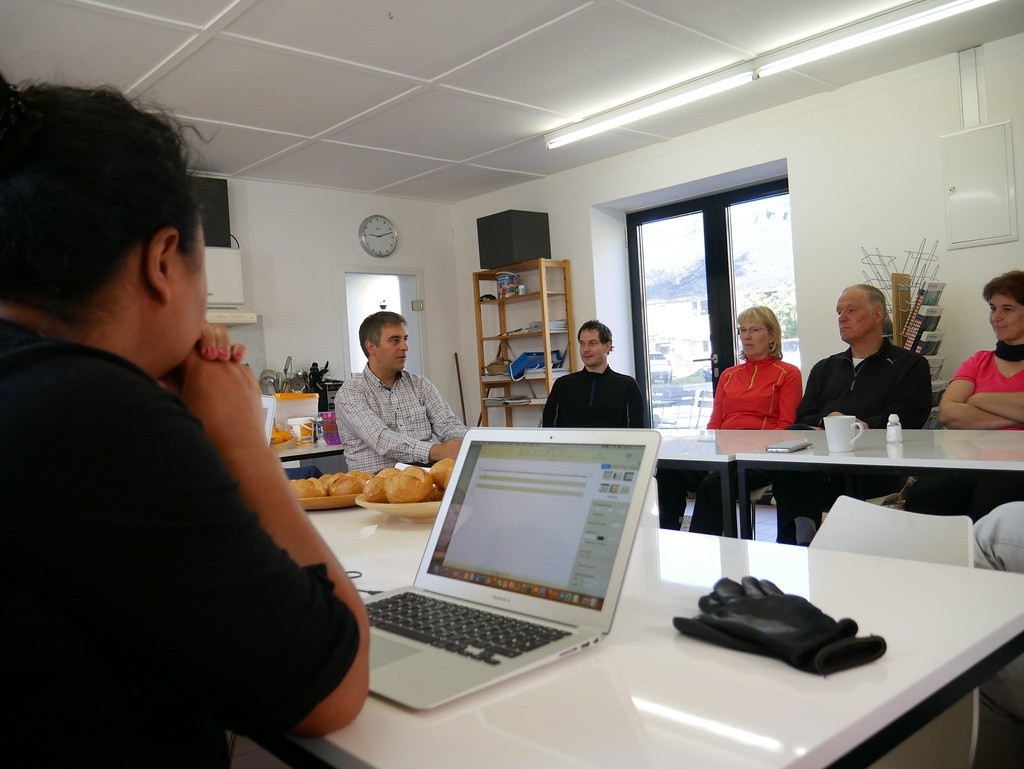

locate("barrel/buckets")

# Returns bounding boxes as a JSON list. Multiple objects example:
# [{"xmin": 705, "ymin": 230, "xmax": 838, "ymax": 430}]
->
[
  {"xmin": 288, "ymin": 417, "xmax": 315, "ymax": 446},
  {"xmin": 495, "ymin": 271, "xmax": 520, "ymax": 299},
  {"xmin": 272, "ymin": 392, "xmax": 318, "ymax": 432}
]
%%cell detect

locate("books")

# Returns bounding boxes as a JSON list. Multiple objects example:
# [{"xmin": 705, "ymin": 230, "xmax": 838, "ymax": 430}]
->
[
  {"xmin": 528, "ymin": 320, "xmax": 567, "ymax": 331},
  {"xmin": 902, "ymin": 281, "xmax": 949, "ymax": 430},
  {"xmin": 482, "ymin": 395, "xmax": 546, "ymax": 404},
  {"xmin": 525, "ymin": 368, "xmax": 570, "ymax": 379},
  {"xmin": 482, "ymin": 374, "xmax": 511, "ymax": 382}
]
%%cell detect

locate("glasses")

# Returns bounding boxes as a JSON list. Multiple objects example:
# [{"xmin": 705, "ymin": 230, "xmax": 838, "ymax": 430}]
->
[{"xmin": 738, "ymin": 326, "xmax": 770, "ymax": 335}]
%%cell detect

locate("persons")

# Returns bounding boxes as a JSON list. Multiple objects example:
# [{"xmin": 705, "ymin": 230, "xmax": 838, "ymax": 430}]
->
[
  {"xmin": 542, "ymin": 321, "xmax": 645, "ymax": 428},
  {"xmin": 656, "ymin": 306, "xmax": 803, "ymax": 537},
  {"xmin": 0, "ymin": 80, "xmax": 370, "ymax": 769},
  {"xmin": 905, "ymin": 271, "xmax": 1024, "ymax": 524},
  {"xmin": 772, "ymin": 284, "xmax": 932, "ymax": 546},
  {"xmin": 334, "ymin": 311, "xmax": 466, "ymax": 474},
  {"xmin": 973, "ymin": 501, "xmax": 1024, "ymax": 730}
]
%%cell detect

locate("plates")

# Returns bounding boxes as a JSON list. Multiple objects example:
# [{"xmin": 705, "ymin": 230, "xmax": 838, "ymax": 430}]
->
[
  {"xmin": 355, "ymin": 495, "xmax": 441, "ymax": 524},
  {"xmin": 297, "ymin": 493, "xmax": 362, "ymax": 510}
]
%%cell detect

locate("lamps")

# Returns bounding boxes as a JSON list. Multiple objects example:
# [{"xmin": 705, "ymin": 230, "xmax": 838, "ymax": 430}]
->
[{"xmin": 542, "ymin": 0, "xmax": 999, "ymax": 147}]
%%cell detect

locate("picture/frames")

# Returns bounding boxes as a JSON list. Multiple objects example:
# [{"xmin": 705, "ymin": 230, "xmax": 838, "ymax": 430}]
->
[{"xmin": 261, "ymin": 393, "xmax": 276, "ymax": 445}]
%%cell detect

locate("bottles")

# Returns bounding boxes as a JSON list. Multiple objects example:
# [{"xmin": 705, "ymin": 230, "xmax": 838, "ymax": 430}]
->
[
  {"xmin": 518, "ymin": 284, "xmax": 525, "ymax": 295},
  {"xmin": 886, "ymin": 414, "xmax": 903, "ymax": 443}
]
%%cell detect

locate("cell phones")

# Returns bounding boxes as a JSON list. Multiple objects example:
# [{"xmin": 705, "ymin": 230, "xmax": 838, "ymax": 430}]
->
[{"xmin": 766, "ymin": 442, "xmax": 811, "ymax": 453}]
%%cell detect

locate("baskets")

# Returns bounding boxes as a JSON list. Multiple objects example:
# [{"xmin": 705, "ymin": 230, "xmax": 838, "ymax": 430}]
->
[{"xmin": 482, "ymin": 363, "xmax": 509, "ymax": 374}]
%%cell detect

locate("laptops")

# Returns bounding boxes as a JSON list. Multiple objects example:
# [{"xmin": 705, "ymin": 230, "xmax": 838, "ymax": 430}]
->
[{"xmin": 353, "ymin": 427, "xmax": 662, "ymax": 710}]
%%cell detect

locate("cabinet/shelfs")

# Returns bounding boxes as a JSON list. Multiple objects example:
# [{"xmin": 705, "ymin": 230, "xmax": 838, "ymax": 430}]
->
[{"xmin": 471, "ymin": 258, "xmax": 575, "ymax": 427}]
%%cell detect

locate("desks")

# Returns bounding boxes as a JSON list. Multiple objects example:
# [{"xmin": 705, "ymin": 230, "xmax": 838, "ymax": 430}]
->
[{"xmin": 230, "ymin": 430, "xmax": 1024, "ymax": 769}]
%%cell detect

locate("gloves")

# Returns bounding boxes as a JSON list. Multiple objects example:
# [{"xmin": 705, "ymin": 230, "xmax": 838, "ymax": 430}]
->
[{"xmin": 673, "ymin": 575, "xmax": 886, "ymax": 676}]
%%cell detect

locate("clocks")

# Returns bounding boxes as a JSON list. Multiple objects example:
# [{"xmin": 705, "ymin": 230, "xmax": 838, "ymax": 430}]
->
[{"xmin": 359, "ymin": 215, "xmax": 398, "ymax": 257}]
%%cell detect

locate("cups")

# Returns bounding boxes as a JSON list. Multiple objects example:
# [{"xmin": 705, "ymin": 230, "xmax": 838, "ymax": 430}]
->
[
  {"xmin": 823, "ymin": 415, "xmax": 864, "ymax": 453},
  {"xmin": 322, "ymin": 412, "xmax": 342, "ymax": 444}
]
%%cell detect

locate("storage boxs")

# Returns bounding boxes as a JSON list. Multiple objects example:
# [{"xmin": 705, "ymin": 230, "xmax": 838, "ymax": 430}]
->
[{"xmin": 274, "ymin": 392, "xmax": 320, "ymax": 425}]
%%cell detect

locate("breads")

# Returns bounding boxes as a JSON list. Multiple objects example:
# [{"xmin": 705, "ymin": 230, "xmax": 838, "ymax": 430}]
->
[
  {"xmin": 272, "ymin": 427, "xmax": 294, "ymax": 445},
  {"xmin": 291, "ymin": 469, "xmax": 377, "ymax": 499},
  {"xmin": 363, "ymin": 457, "xmax": 454, "ymax": 505}
]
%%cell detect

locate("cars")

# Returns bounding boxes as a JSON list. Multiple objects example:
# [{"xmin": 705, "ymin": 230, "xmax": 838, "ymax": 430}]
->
[{"xmin": 648, "ymin": 352, "xmax": 673, "ymax": 384}]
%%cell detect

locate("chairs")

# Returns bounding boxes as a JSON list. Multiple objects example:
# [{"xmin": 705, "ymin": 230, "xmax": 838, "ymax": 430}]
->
[{"xmin": 809, "ymin": 494, "xmax": 979, "ymax": 769}]
